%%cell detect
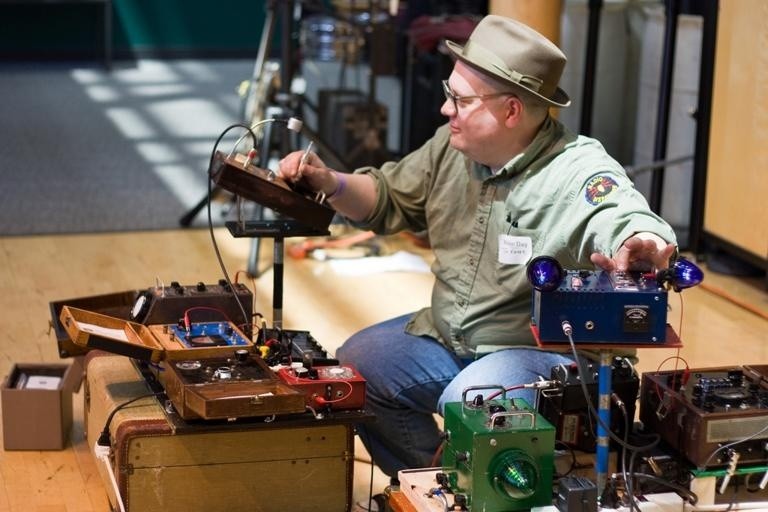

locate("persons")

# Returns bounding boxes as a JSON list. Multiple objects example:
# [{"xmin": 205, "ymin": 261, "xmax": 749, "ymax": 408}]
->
[{"xmin": 275, "ymin": 12, "xmax": 679, "ymax": 512}]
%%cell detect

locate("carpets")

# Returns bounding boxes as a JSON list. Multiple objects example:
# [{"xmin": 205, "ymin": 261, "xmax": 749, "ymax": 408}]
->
[{"xmin": 1, "ymin": 60, "xmax": 402, "ymax": 236}]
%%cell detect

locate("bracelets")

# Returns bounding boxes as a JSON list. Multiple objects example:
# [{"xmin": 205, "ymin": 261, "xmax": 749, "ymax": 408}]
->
[{"xmin": 326, "ymin": 170, "xmax": 346, "ymax": 202}]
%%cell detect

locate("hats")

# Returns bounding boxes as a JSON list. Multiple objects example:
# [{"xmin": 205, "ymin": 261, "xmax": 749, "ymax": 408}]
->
[{"xmin": 439, "ymin": 12, "xmax": 574, "ymax": 112}]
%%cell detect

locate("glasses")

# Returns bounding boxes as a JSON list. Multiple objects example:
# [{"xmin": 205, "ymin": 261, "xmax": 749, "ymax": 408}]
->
[{"xmin": 442, "ymin": 78, "xmax": 519, "ymax": 112}]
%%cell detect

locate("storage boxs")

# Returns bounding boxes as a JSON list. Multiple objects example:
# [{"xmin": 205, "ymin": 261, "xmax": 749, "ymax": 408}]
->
[
  {"xmin": 82, "ymin": 347, "xmax": 355, "ymax": 512},
  {"xmin": 1, "ymin": 363, "xmax": 83, "ymax": 451}
]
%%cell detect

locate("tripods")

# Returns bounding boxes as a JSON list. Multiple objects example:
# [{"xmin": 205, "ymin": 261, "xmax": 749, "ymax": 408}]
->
[{"xmin": 179, "ymin": 0, "xmax": 353, "ymax": 278}]
